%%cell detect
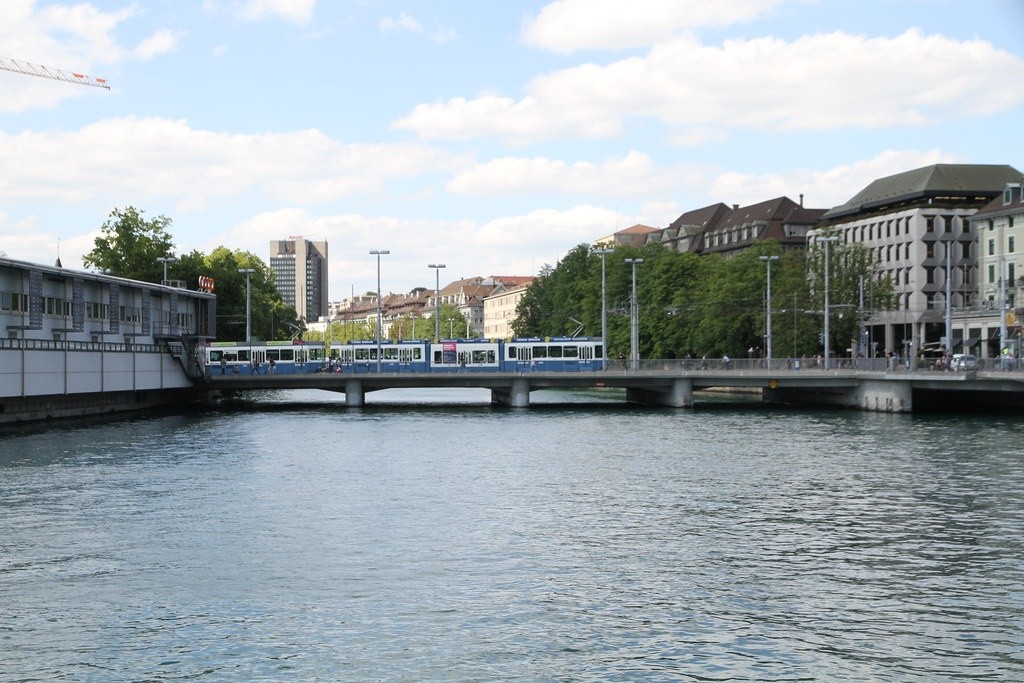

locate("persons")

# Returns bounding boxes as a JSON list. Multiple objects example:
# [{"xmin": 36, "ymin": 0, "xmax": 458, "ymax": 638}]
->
[
  {"xmin": 458, "ymin": 359, "xmax": 467, "ymax": 373},
  {"xmin": 220, "ymin": 357, "xmax": 227, "ymax": 373},
  {"xmin": 268, "ymin": 358, "xmax": 276, "ymax": 375},
  {"xmin": 251, "ymin": 360, "xmax": 262, "ymax": 375},
  {"xmin": 615, "ymin": 348, "xmax": 1014, "ymax": 373},
  {"xmin": 327, "ymin": 357, "xmax": 343, "ymax": 373}
]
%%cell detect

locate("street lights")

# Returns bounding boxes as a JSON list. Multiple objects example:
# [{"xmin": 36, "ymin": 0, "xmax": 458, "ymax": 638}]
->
[
  {"xmin": 369, "ymin": 250, "xmax": 391, "ymax": 375},
  {"xmin": 757, "ymin": 255, "xmax": 781, "ymax": 369},
  {"xmin": 816, "ymin": 233, "xmax": 840, "ymax": 371},
  {"xmin": 591, "ymin": 248, "xmax": 615, "ymax": 372},
  {"xmin": 624, "ymin": 258, "xmax": 644, "ymax": 370},
  {"xmin": 156, "ymin": 256, "xmax": 175, "ymax": 284},
  {"xmin": 238, "ymin": 268, "xmax": 257, "ymax": 342},
  {"xmin": 428, "ymin": 262, "xmax": 447, "ymax": 337}
]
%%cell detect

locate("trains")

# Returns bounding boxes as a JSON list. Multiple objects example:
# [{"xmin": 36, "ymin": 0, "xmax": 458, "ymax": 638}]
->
[{"xmin": 204, "ymin": 335, "xmax": 610, "ymax": 374}]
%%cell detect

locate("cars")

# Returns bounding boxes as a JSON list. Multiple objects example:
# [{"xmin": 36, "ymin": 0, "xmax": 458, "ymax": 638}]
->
[{"xmin": 951, "ymin": 353, "xmax": 979, "ymax": 371}]
